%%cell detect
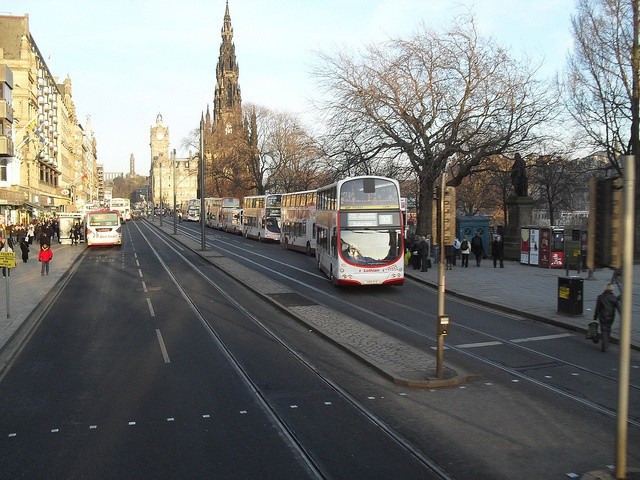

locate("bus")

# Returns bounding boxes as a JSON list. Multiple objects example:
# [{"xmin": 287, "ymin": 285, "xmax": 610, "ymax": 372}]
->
[
  {"xmin": 281, "ymin": 190, "xmax": 316, "ymax": 257},
  {"xmin": 316, "ymin": 175, "xmax": 405, "ymax": 287},
  {"xmin": 181, "ymin": 199, "xmax": 200, "ymax": 221},
  {"xmin": 204, "ymin": 197, "xmax": 240, "ymax": 232},
  {"xmin": 243, "ymin": 194, "xmax": 281, "ymax": 242},
  {"xmin": 87, "ymin": 210, "xmax": 122, "ymax": 249},
  {"xmin": 110, "ymin": 198, "xmax": 130, "ymax": 221}
]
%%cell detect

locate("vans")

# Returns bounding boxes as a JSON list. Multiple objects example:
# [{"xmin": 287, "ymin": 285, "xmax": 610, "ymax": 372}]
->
[{"xmin": 222, "ymin": 209, "xmax": 242, "ymax": 235}]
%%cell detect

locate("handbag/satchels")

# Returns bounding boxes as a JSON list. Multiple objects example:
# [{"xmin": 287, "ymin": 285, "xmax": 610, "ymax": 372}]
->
[
  {"xmin": 482, "ymin": 251, "xmax": 488, "ymax": 260},
  {"xmin": 587, "ymin": 322, "xmax": 599, "ymax": 343}
]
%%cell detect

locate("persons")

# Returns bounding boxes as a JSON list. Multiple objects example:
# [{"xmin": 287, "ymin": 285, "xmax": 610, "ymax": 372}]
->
[
  {"xmin": 424, "ymin": 233, "xmax": 432, "ymax": 267},
  {"xmin": 177, "ymin": 213, "xmax": 183, "ymax": 225},
  {"xmin": 1, "ymin": 242, "xmax": 13, "ymax": 278},
  {"xmin": 70, "ymin": 222, "xmax": 87, "ymax": 246},
  {"xmin": 26, "ymin": 227, "xmax": 34, "ymax": 245},
  {"xmin": 461, "ymin": 234, "xmax": 471, "ymax": 268},
  {"xmin": 39, "ymin": 241, "xmax": 53, "ymax": 276},
  {"xmin": 453, "ymin": 237, "xmax": 460, "ymax": 264},
  {"xmin": 593, "ymin": 283, "xmax": 623, "ymax": 353},
  {"xmin": 413, "ymin": 236, "xmax": 429, "ymax": 272},
  {"xmin": 408, "ymin": 234, "xmax": 422, "ymax": 270},
  {"xmin": 8, "ymin": 223, "xmax": 25, "ymax": 248},
  {"xmin": 403, "ymin": 227, "xmax": 412, "ymax": 267},
  {"xmin": 35, "ymin": 218, "xmax": 59, "ymax": 243},
  {"xmin": 470, "ymin": 231, "xmax": 488, "ymax": 268},
  {"xmin": 492, "ymin": 235, "xmax": 504, "ymax": 268},
  {"xmin": 20, "ymin": 237, "xmax": 30, "ymax": 263}
]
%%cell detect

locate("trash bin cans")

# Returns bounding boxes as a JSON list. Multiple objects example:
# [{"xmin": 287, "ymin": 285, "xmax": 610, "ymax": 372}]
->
[{"xmin": 557, "ymin": 275, "xmax": 584, "ymax": 318}]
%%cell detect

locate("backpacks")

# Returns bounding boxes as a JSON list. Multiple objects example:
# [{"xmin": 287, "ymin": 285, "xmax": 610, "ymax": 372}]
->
[{"xmin": 461, "ymin": 240, "xmax": 468, "ymax": 249}]
